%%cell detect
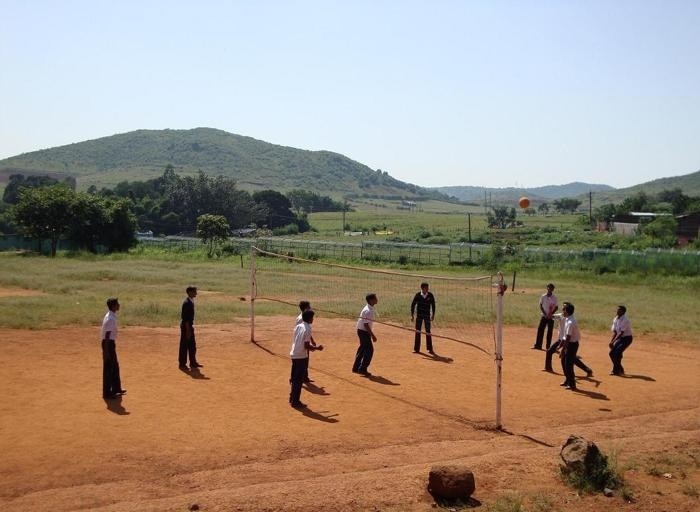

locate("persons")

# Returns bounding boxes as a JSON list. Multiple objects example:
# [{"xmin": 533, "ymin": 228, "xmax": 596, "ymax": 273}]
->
[
  {"xmin": 542, "ymin": 302, "xmax": 593, "ymax": 378},
  {"xmin": 608, "ymin": 305, "xmax": 633, "ymax": 375},
  {"xmin": 410, "ymin": 282, "xmax": 437, "ymax": 353},
  {"xmin": 556, "ymin": 303, "xmax": 583, "ymax": 390},
  {"xmin": 291, "ymin": 300, "xmax": 316, "ymax": 383},
  {"xmin": 288, "ymin": 309, "xmax": 325, "ymax": 408},
  {"xmin": 529, "ymin": 283, "xmax": 560, "ymax": 352},
  {"xmin": 100, "ymin": 296, "xmax": 128, "ymax": 399},
  {"xmin": 177, "ymin": 286, "xmax": 204, "ymax": 369},
  {"xmin": 351, "ymin": 293, "xmax": 380, "ymax": 377}
]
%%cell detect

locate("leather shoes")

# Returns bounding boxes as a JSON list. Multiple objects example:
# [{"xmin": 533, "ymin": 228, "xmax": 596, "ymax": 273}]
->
[
  {"xmin": 586, "ymin": 367, "xmax": 593, "ymax": 378},
  {"xmin": 412, "ymin": 350, "xmax": 433, "ymax": 354},
  {"xmin": 352, "ymin": 366, "xmax": 371, "ymax": 377},
  {"xmin": 178, "ymin": 363, "xmax": 203, "ymax": 370},
  {"xmin": 559, "ymin": 382, "xmax": 577, "ymax": 390},
  {"xmin": 103, "ymin": 388, "xmax": 127, "ymax": 399}
]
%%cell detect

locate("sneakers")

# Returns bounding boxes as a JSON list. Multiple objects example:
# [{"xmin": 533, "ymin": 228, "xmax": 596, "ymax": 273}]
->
[
  {"xmin": 609, "ymin": 368, "xmax": 625, "ymax": 376},
  {"xmin": 288, "ymin": 397, "xmax": 307, "ymax": 410},
  {"xmin": 530, "ymin": 346, "xmax": 555, "ymax": 372}
]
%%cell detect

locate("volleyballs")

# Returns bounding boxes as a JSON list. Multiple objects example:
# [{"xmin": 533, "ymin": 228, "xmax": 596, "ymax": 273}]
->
[{"xmin": 519, "ymin": 197, "xmax": 530, "ymax": 208}]
